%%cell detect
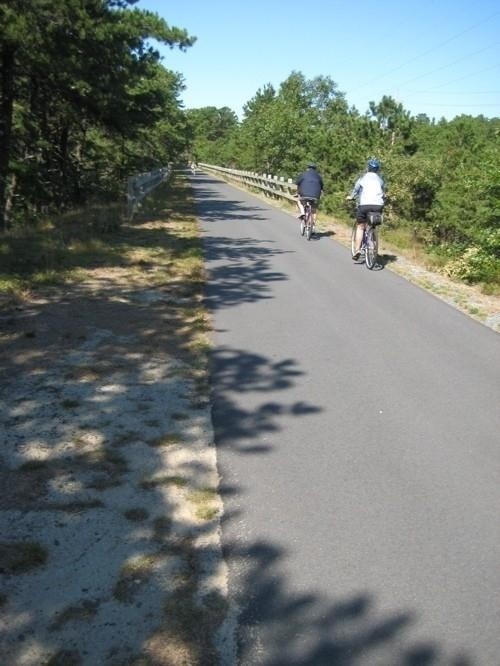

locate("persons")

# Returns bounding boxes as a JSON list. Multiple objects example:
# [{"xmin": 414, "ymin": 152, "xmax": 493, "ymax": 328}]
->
[
  {"xmin": 295, "ymin": 162, "xmax": 323, "ymax": 226},
  {"xmin": 346, "ymin": 160, "xmax": 385, "ymax": 260}
]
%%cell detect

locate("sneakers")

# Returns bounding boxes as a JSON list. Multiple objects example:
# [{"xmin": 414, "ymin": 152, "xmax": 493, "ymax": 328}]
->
[
  {"xmin": 352, "ymin": 251, "xmax": 361, "ymax": 260},
  {"xmin": 296, "ymin": 213, "xmax": 306, "ymax": 218}
]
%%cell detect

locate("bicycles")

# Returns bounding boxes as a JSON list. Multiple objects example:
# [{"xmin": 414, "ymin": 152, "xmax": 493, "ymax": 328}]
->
[
  {"xmin": 345, "ymin": 197, "xmax": 382, "ymax": 270},
  {"xmin": 294, "ymin": 192, "xmax": 317, "ymax": 241},
  {"xmin": 191, "ymin": 166, "xmax": 198, "ymax": 177}
]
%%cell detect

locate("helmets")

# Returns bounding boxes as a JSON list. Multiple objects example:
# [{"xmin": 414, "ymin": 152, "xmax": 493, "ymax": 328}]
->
[
  {"xmin": 367, "ymin": 159, "xmax": 379, "ymax": 170},
  {"xmin": 307, "ymin": 164, "xmax": 317, "ymax": 170}
]
